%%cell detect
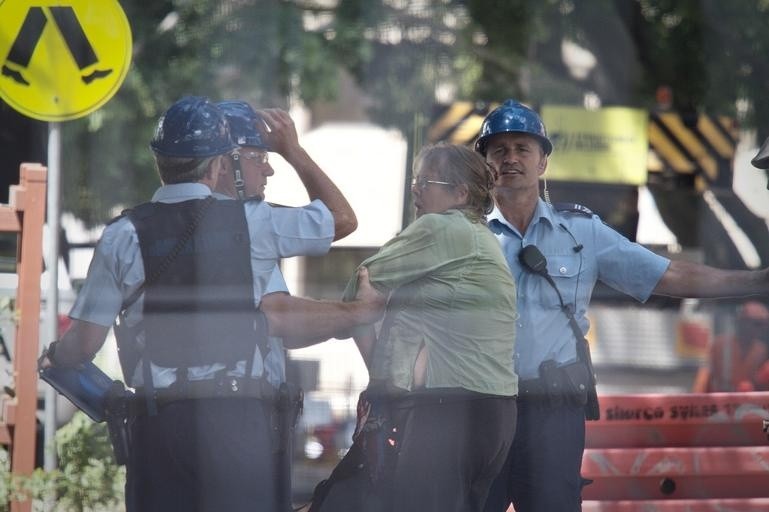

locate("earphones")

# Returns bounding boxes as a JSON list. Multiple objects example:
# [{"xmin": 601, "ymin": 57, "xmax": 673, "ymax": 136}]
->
[{"xmin": 540, "ymin": 162, "xmax": 545, "ymax": 168}]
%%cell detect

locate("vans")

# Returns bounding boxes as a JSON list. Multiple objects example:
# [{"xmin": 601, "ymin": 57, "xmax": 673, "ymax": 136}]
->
[{"xmin": 1, "ymin": 231, "xmax": 83, "ymax": 476}]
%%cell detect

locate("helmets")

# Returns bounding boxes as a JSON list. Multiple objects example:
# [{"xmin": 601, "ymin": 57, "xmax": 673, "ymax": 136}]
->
[
  {"xmin": 474, "ymin": 99, "xmax": 552, "ymax": 156},
  {"xmin": 149, "ymin": 96, "xmax": 270, "ymax": 158}
]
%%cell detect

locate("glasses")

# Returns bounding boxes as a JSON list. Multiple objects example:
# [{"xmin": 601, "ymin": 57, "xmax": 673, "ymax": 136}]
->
[
  {"xmin": 240, "ymin": 151, "xmax": 268, "ymax": 166},
  {"xmin": 416, "ymin": 177, "xmax": 456, "ymax": 190}
]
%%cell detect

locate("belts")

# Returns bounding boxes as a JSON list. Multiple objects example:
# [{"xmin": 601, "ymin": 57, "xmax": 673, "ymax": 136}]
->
[
  {"xmin": 138, "ymin": 375, "xmax": 280, "ymax": 411},
  {"xmin": 372, "ymin": 391, "xmax": 500, "ymax": 408}
]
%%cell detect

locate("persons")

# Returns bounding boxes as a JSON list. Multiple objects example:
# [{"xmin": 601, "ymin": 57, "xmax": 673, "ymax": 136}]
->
[
  {"xmin": 214, "ymin": 102, "xmax": 389, "ymax": 511},
  {"xmin": 311, "ymin": 141, "xmax": 520, "ymax": 510},
  {"xmin": 37, "ymin": 98, "xmax": 358, "ymax": 511},
  {"xmin": 474, "ymin": 99, "xmax": 769, "ymax": 510}
]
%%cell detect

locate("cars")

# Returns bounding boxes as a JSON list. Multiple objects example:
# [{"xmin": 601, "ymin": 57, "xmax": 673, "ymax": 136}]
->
[{"xmin": 290, "ymin": 392, "xmax": 349, "ymax": 466}]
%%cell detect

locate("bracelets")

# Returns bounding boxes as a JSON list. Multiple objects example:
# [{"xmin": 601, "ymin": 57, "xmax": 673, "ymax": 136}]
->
[{"xmin": 46, "ymin": 341, "xmax": 59, "ymax": 366}]
